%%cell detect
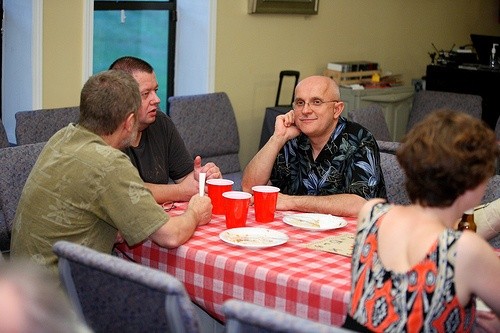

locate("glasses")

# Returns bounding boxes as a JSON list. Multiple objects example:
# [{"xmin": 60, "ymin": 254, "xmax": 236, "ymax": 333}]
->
[{"xmin": 292, "ymin": 98, "xmax": 339, "ymax": 108}]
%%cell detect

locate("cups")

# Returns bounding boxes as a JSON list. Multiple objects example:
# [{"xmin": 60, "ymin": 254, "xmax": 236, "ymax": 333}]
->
[
  {"xmin": 252, "ymin": 186, "xmax": 280, "ymax": 222},
  {"xmin": 221, "ymin": 191, "xmax": 251, "ymax": 229},
  {"xmin": 205, "ymin": 179, "xmax": 234, "ymax": 215}
]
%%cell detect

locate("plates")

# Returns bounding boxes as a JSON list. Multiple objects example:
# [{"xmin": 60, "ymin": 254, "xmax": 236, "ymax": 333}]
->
[
  {"xmin": 219, "ymin": 227, "xmax": 289, "ymax": 248},
  {"xmin": 282, "ymin": 213, "xmax": 348, "ymax": 231}
]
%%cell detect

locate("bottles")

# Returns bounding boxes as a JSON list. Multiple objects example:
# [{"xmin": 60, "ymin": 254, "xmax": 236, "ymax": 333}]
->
[{"xmin": 458, "ymin": 213, "xmax": 477, "ymax": 232}]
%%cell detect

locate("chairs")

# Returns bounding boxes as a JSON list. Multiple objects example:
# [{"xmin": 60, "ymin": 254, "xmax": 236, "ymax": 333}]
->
[{"xmin": 0, "ymin": 90, "xmax": 500, "ymax": 333}]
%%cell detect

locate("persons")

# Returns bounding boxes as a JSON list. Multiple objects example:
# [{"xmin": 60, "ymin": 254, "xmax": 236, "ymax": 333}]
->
[
  {"xmin": 339, "ymin": 111, "xmax": 500, "ymax": 333},
  {"xmin": 10, "ymin": 69, "xmax": 213, "ymax": 290},
  {"xmin": 241, "ymin": 75, "xmax": 389, "ymax": 218},
  {"xmin": 108, "ymin": 56, "xmax": 224, "ymax": 204}
]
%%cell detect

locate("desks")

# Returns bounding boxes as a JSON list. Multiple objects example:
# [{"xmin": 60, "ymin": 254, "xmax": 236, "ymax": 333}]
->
[
  {"xmin": 259, "ymin": 107, "xmax": 293, "ymax": 151},
  {"xmin": 340, "ymin": 85, "xmax": 414, "ymax": 143},
  {"xmin": 113, "ymin": 201, "xmax": 500, "ymax": 333},
  {"xmin": 426, "ymin": 64, "xmax": 500, "ymax": 130}
]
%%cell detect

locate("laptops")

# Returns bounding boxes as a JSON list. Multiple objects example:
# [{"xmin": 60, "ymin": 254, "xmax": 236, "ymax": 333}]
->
[{"xmin": 470, "ymin": 33, "xmax": 500, "ymax": 68}]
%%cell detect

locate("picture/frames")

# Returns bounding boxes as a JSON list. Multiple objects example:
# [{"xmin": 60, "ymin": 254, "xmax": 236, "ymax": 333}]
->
[{"xmin": 248, "ymin": 0, "xmax": 319, "ymax": 15}]
